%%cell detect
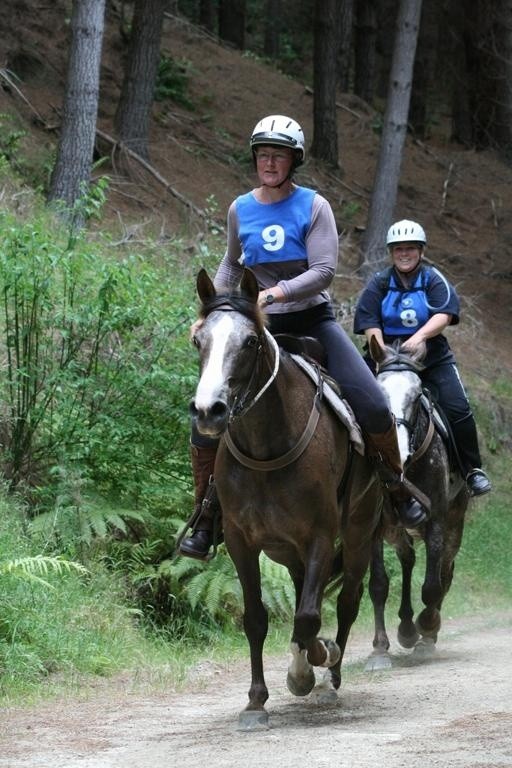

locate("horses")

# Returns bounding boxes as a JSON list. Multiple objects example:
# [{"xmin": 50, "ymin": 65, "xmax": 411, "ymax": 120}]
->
[
  {"xmin": 180, "ymin": 262, "xmax": 395, "ymax": 731},
  {"xmin": 357, "ymin": 334, "xmax": 472, "ymax": 678}
]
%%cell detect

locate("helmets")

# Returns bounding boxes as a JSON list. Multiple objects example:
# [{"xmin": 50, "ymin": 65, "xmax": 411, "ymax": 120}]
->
[
  {"xmin": 385, "ymin": 219, "xmax": 426, "ymax": 247},
  {"xmin": 248, "ymin": 114, "xmax": 306, "ymax": 159}
]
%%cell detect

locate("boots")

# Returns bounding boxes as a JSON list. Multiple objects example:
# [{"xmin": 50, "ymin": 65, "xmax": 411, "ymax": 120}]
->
[
  {"xmin": 451, "ymin": 412, "xmax": 493, "ymax": 495},
  {"xmin": 369, "ymin": 406, "xmax": 430, "ymax": 527},
  {"xmin": 179, "ymin": 436, "xmax": 227, "ymax": 561}
]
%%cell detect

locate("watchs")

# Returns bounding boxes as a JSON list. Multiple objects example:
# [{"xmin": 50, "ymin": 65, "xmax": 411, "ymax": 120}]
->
[{"xmin": 262, "ymin": 289, "xmax": 274, "ymax": 306}]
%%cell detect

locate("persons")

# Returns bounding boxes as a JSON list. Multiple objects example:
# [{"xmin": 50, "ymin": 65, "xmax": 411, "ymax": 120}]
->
[
  {"xmin": 179, "ymin": 114, "xmax": 427, "ymax": 558},
  {"xmin": 352, "ymin": 218, "xmax": 492, "ymax": 495}
]
